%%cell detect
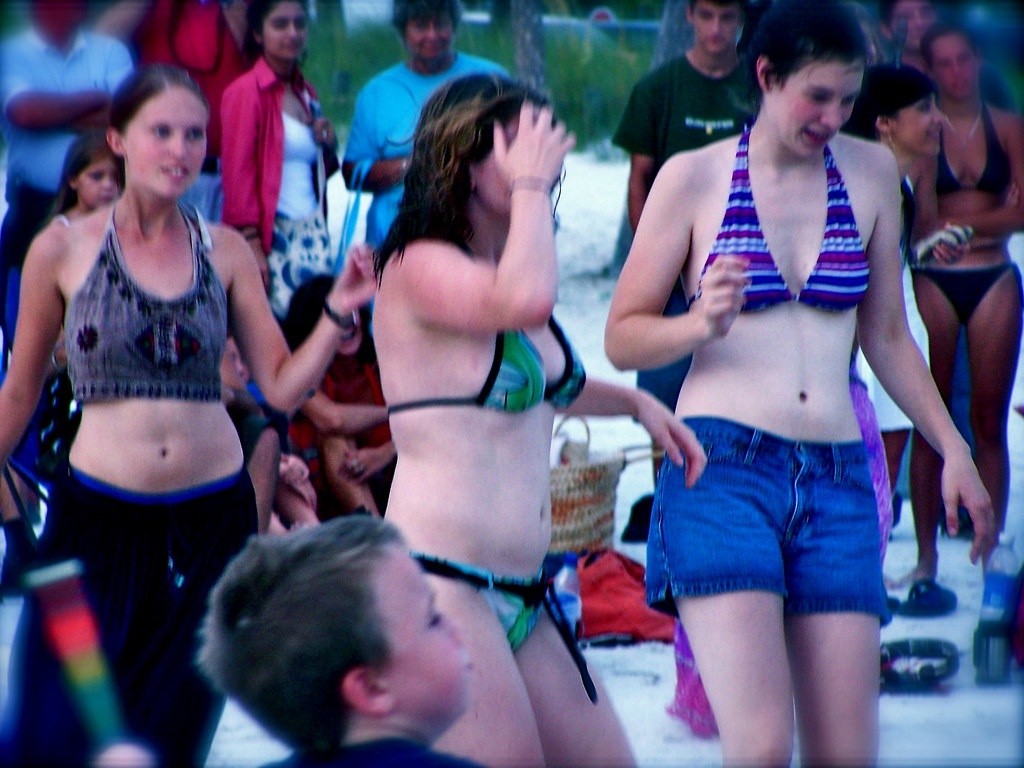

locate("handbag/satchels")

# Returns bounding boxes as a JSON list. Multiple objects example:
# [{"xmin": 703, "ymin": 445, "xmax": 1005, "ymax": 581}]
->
[
  {"xmin": 545, "ymin": 414, "xmax": 665, "ymax": 556},
  {"xmin": 575, "ymin": 549, "xmax": 677, "ymax": 639}
]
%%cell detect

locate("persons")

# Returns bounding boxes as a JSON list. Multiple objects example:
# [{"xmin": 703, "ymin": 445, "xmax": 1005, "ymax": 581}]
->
[
  {"xmin": 604, "ymin": 0, "xmax": 1024, "ymax": 767},
  {"xmin": 345, "ymin": 1, "xmax": 515, "ymax": 326},
  {"xmin": 369, "ymin": 74, "xmax": 705, "ymax": 767},
  {"xmin": 0, "ymin": 1, "xmax": 705, "ymax": 267},
  {"xmin": 197, "ymin": 516, "xmax": 490, "ymax": 768},
  {"xmin": 0, "ymin": 60, "xmax": 381, "ymax": 768},
  {"xmin": 218, "ymin": 1, "xmax": 340, "ymax": 320}
]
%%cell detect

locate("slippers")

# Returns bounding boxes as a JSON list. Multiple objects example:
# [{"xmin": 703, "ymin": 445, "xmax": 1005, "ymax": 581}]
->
[{"xmin": 897, "ymin": 577, "xmax": 956, "ymax": 616}]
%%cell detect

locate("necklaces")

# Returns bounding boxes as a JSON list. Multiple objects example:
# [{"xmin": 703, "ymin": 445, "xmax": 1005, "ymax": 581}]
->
[{"xmin": 940, "ymin": 108, "xmax": 981, "ymax": 149}]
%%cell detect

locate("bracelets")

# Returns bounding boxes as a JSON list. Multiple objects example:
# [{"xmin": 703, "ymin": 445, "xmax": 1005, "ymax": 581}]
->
[
  {"xmin": 244, "ymin": 232, "xmax": 259, "ymax": 241},
  {"xmin": 323, "ymin": 298, "xmax": 353, "ymax": 327}
]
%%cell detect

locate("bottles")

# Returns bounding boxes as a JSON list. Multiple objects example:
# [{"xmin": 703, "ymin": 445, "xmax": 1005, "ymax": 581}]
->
[
  {"xmin": 910, "ymin": 225, "xmax": 974, "ymax": 264},
  {"xmin": 546, "ymin": 552, "xmax": 579, "ymax": 642}
]
